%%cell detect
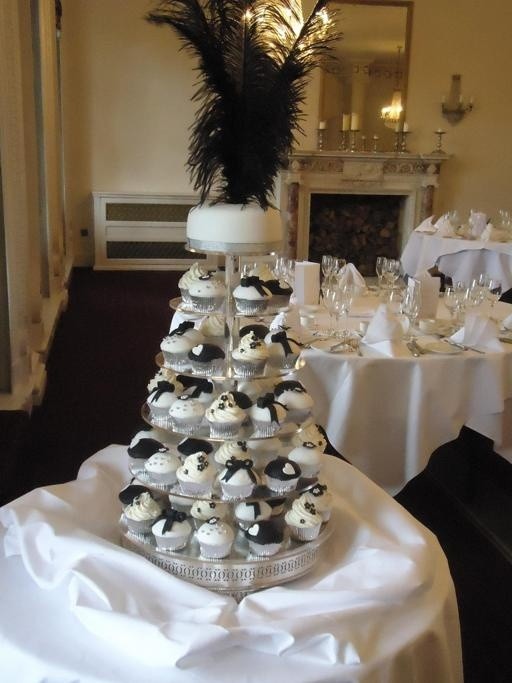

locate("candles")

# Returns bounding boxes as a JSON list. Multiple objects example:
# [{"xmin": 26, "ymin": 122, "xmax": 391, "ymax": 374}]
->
[
  {"xmin": 319, "ymin": 118, "xmax": 325, "ymax": 129},
  {"xmin": 403, "ymin": 120, "xmax": 408, "ymax": 130},
  {"xmin": 351, "ymin": 110, "xmax": 359, "ymax": 130},
  {"xmin": 342, "ymin": 112, "xmax": 350, "ymax": 131},
  {"xmin": 395, "ymin": 122, "xmax": 401, "ymax": 131}
]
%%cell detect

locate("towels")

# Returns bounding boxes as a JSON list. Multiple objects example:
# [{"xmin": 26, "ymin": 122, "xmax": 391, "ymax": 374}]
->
[
  {"xmin": 445, "ymin": 312, "xmax": 501, "ymax": 354},
  {"xmin": 337, "ymin": 262, "xmax": 370, "ymax": 295},
  {"xmin": 355, "ymin": 301, "xmax": 403, "ymax": 356},
  {"xmin": 415, "ymin": 212, "xmax": 501, "ymax": 242}
]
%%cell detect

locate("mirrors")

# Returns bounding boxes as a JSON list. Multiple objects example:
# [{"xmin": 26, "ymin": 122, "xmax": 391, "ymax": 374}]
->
[{"xmin": 314, "ymin": 0, "xmax": 412, "ymax": 153}]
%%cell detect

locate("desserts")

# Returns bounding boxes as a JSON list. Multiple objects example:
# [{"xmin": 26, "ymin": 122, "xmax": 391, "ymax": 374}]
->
[{"xmin": 117, "ymin": 261, "xmax": 334, "ymax": 559}]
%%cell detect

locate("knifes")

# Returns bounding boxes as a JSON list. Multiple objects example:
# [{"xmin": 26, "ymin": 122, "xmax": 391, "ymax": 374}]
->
[{"xmin": 407, "ymin": 339, "xmax": 424, "ymax": 356}]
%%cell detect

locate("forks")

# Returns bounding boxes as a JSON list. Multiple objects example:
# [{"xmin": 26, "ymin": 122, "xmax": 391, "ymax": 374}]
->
[{"xmin": 350, "ymin": 339, "xmax": 363, "ymax": 356}]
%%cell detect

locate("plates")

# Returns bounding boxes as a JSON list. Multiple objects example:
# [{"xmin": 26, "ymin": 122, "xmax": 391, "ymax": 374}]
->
[
  {"xmin": 307, "ymin": 341, "xmax": 348, "ymax": 354},
  {"xmin": 417, "ymin": 337, "xmax": 466, "ymax": 353}
]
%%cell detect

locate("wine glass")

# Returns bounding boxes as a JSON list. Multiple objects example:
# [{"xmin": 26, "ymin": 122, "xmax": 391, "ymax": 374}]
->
[{"xmin": 242, "ymin": 255, "xmax": 503, "ymax": 343}]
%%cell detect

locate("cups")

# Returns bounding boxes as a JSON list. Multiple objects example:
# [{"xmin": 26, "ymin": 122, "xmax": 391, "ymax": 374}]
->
[{"xmin": 442, "ymin": 206, "xmax": 511, "ymax": 239}]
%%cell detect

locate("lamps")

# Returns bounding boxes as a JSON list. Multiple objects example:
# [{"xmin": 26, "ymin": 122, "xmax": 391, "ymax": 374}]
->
[{"xmin": 378, "ymin": 42, "xmax": 403, "ymax": 124}]
